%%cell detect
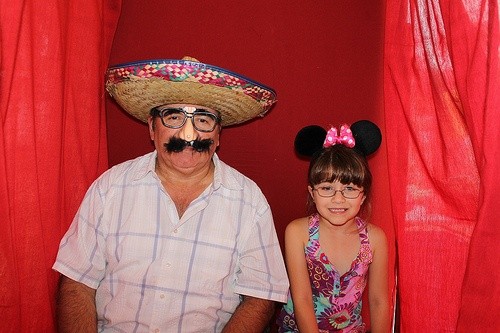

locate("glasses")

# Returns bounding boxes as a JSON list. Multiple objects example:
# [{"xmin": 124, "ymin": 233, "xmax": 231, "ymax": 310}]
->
[
  {"xmin": 152, "ymin": 107, "xmax": 220, "ymax": 133},
  {"xmin": 312, "ymin": 186, "xmax": 364, "ymax": 199}
]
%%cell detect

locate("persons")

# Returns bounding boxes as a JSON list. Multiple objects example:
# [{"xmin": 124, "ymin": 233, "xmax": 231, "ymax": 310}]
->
[
  {"xmin": 51, "ymin": 55, "xmax": 291, "ymax": 333},
  {"xmin": 273, "ymin": 120, "xmax": 392, "ymax": 333}
]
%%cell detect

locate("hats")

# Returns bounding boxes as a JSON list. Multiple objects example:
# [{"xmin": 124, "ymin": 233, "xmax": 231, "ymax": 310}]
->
[{"xmin": 105, "ymin": 55, "xmax": 278, "ymax": 127}]
